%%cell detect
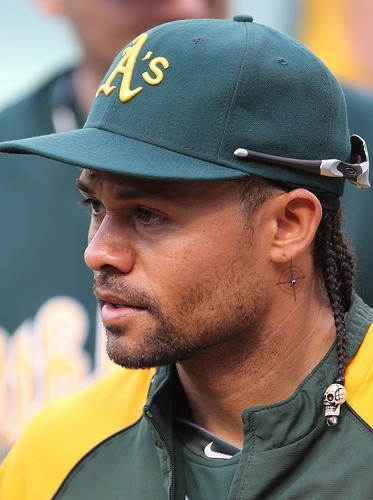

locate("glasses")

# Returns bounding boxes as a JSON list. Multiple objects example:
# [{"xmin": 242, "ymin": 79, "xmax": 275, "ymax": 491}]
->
[{"xmin": 234, "ymin": 133, "xmax": 371, "ymax": 189}]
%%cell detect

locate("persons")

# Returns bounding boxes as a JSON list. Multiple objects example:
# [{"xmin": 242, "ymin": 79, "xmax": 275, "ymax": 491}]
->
[
  {"xmin": 0, "ymin": 15, "xmax": 373, "ymax": 500},
  {"xmin": 0, "ymin": 0, "xmax": 373, "ymax": 463}
]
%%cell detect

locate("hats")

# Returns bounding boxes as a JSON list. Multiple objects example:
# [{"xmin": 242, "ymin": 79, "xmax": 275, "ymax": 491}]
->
[{"xmin": 0, "ymin": 15, "xmax": 351, "ymax": 197}]
